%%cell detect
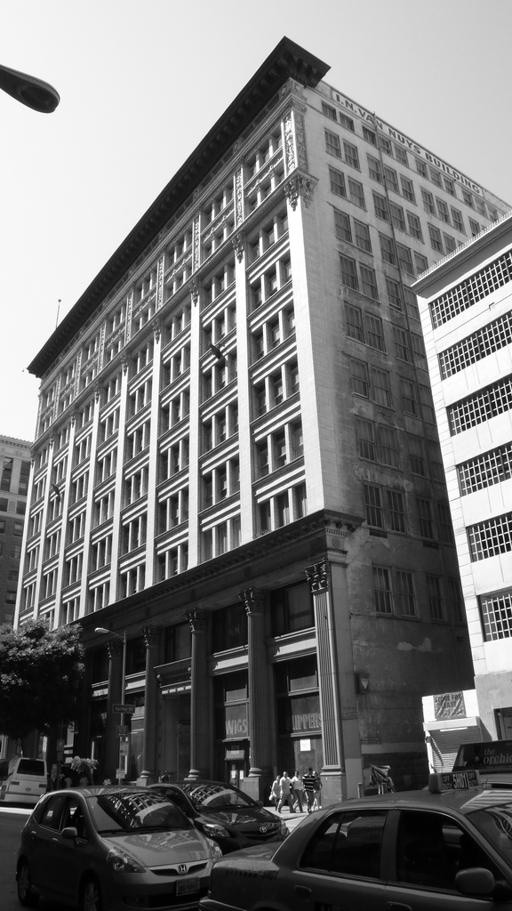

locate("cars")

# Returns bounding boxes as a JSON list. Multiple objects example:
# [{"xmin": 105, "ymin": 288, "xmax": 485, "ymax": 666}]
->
[
  {"xmin": 199, "ymin": 770, "xmax": 511, "ymax": 911},
  {"xmin": 15, "ymin": 786, "xmax": 224, "ymax": 911},
  {"xmin": 146, "ymin": 780, "xmax": 290, "ymax": 856}
]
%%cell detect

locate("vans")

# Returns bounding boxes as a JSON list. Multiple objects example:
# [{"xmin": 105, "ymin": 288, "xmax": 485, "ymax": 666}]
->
[{"xmin": 0, "ymin": 757, "xmax": 48, "ymax": 808}]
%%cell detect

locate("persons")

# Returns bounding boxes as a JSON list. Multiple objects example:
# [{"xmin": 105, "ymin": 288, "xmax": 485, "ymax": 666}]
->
[
  {"xmin": 302, "ymin": 765, "xmax": 320, "ymax": 813},
  {"xmin": 267, "ymin": 774, "xmax": 280, "ymax": 807},
  {"xmin": 291, "ymin": 767, "xmax": 305, "ymax": 814},
  {"xmin": 278, "ymin": 769, "xmax": 296, "ymax": 814}
]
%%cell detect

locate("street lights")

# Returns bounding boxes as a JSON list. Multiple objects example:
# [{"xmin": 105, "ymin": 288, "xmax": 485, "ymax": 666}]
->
[
  {"xmin": 95, "ymin": 627, "xmax": 128, "ymax": 785},
  {"xmin": 1, "ymin": 65, "xmax": 61, "ymax": 114}
]
